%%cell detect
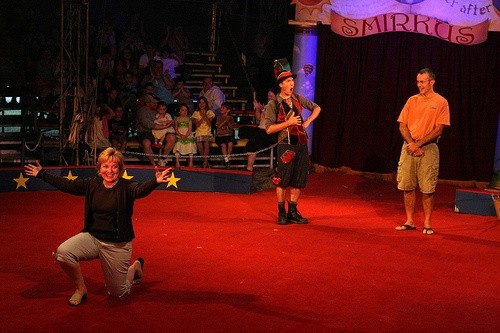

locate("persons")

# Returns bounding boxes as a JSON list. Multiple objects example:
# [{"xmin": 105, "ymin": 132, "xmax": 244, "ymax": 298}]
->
[
  {"xmin": 264, "ymin": 69, "xmax": 321, "ymax": 225},
  {"xmin": 395, "ymin": 67, "xmax": 450, "ymax": 235},
  {"xmin": 91, "ymin": 13, "xmax": 276, "ymax": 172},
  {"xmin": 24, "ymin": 147, "xmax": 172, "ymax": 307}
]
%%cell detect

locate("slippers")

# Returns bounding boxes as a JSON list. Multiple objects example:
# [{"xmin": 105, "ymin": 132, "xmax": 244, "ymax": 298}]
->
[
  {"xmin": 68, "ymin": 288, "xmax": 87, "ymax": 306},
  {"xmin": 422, "ymin": 228, "xmax": 434, "ymax": 235},
  {"xmin": 132, "ymin": 257, "xmax": 144, "ymax": 288},
  {"xmin": 395, "ymin": 224, "xmax": 416, "ymax": 231}
]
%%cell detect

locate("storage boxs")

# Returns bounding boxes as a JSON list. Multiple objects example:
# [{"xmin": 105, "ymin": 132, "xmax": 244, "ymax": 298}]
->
[{"xmin": 455, "ymin": 187, "xmax": 500, "ymax": 216}]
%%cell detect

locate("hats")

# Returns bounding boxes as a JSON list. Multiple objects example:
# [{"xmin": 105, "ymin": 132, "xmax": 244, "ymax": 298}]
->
[{"xmin": 272, "ymin": 59, "xmax": 297, "ymax": 84}]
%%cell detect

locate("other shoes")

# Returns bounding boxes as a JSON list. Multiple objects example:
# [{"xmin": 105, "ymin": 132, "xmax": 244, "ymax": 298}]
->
[{"xmin": 224, "ymin": 160, "xmax": 232, "ymax": 168}]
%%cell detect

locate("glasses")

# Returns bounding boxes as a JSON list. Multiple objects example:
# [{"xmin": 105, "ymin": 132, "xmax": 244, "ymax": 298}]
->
[{"xmin": 416, "ymin": 80, "xmax": 430, "ymax": 83}]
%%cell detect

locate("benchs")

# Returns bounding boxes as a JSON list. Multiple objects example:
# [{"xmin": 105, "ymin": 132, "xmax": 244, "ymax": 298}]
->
[{"xmin": 117, "ymin": 53, "xmax": 277, "ymax": 170}]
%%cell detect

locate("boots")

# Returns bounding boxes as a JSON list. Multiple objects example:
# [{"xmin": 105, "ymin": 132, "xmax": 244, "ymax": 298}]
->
[
  {"xmin": 277, "ymin": 201, "xmax": 287, "ymax": 224},
  {"xmin": 287, "ymin": 200, "xmax": 308, "ymax": 224}
]
